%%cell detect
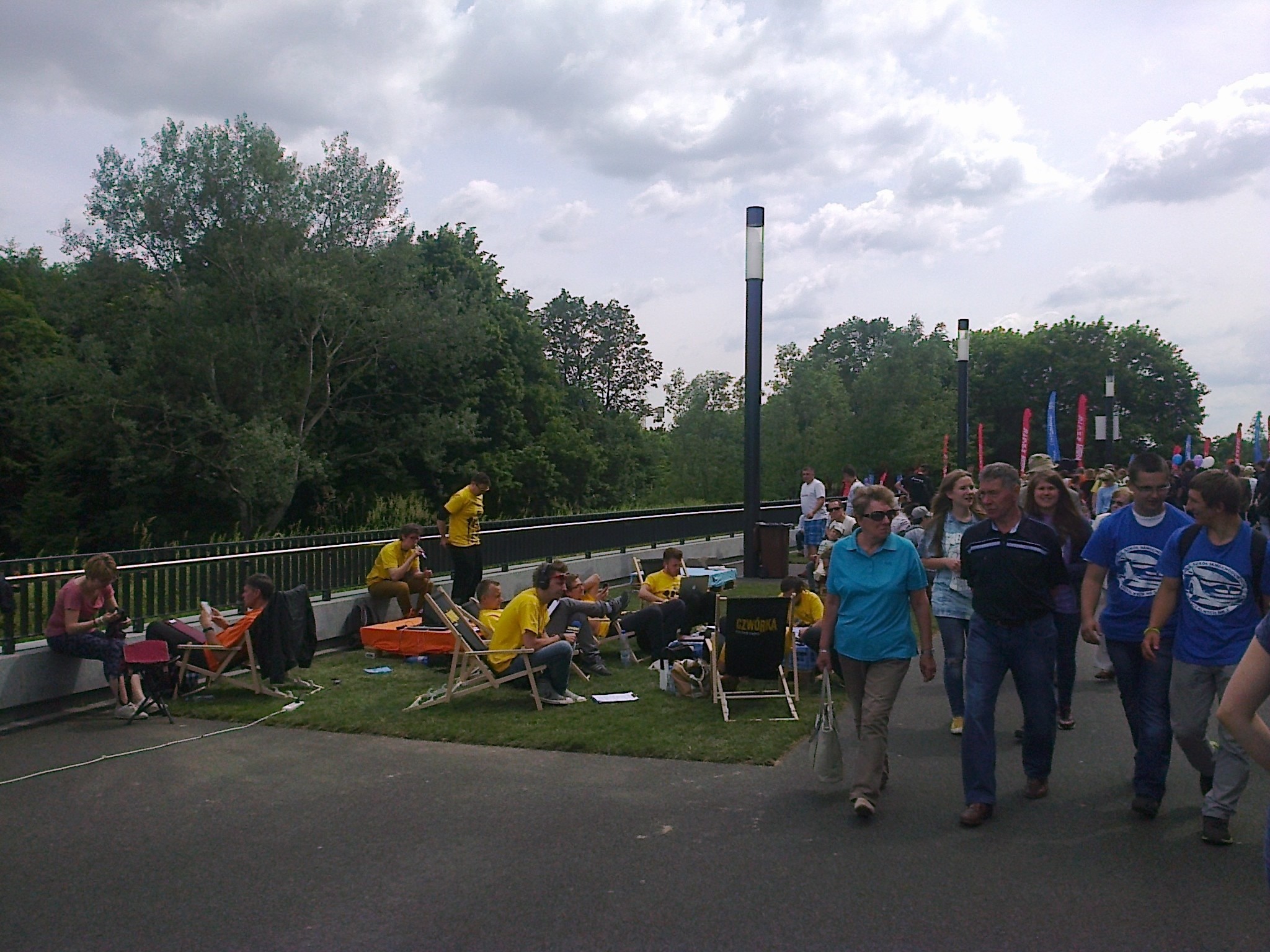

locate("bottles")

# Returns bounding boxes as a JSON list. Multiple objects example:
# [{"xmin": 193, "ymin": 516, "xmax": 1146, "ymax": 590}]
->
[
  {"xmin": 620, "ymin": 630, "xmax": 631, "ymax": 667},
  {"xmin": 405, "ymin": 656, "xmax": 428, "ymax": 664},
  {"xmin": 667, "ymin": 666, "xmax": 676, "ymax": 695},
  {"xmin": 415, "ymin": 545, "xmax": 427, "ymax": 559}
]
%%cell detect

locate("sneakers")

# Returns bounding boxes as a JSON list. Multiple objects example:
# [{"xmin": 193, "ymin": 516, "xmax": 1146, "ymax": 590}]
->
[
  {"xmin": 1202, "ymin": 815, "xmax": 1232, "ymax": 845},
  {"xmin": 138, "ymin": 698, "xmax": 168, "ymax": 713},
  {"xmin": 1200, "ymin": 741, "xmax": 1219, "ymax": 797},
  {"xmin": 606, "ymin": 592, "xmax": 630, "ymax": 622},
  {"xmin": 584, "ymin": 661, "xmax": 612, "ymax": 677},
  {"xmin": 114, "ymin": 702, "xmax": 149, "ymax": 719}
]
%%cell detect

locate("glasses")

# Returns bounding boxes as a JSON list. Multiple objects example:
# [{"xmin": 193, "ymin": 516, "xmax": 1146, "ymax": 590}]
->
[
  {"xmin": 827, "ymin": 507, "xmax": 842, "ymax": 512},
  {"xmin": 863, "ymin": 509, "xmax": 896, "ymax": 521},
  {"xmin": 1130, "ymin": 479, "xmax": 1171, "ymax": 493}
]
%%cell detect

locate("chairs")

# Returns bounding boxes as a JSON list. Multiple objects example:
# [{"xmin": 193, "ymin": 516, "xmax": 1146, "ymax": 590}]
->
[
  {"xmin": 402, "ymin": 557, "xmax": 800, "ymax": 722},
  {"xmin": 174, "ymin": 584, "xmax": 324, "ymax": 700}
]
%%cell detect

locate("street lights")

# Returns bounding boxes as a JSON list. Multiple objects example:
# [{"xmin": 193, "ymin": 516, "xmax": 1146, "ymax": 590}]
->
[
  {"xmin": 1104, "ymin": 368, "xmax": 1117, "ymax": 464},
  {"xmin": 956, "ymin": 318, "xmax": 970, "ymax": 471},
  {"xmin": 742, "ymin": 205, "xmax": 765, "ymax": 578}
]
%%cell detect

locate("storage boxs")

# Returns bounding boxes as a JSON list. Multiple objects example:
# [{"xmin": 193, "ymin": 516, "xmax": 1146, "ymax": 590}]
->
[{"xmin": 789, "ymin": 646, "xmax": 817, "ymax": 670}]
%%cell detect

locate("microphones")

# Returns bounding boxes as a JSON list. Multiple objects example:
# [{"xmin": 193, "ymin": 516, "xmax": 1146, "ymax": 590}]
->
[{"xmin": 415, "ymin": 544, "xmax": 427, "ymax": 559}]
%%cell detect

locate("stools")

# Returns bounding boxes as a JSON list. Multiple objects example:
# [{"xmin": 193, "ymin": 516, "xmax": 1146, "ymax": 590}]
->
[{"xmin": 123, "ymin": 640, "xmax": 180, "ymax": 723}]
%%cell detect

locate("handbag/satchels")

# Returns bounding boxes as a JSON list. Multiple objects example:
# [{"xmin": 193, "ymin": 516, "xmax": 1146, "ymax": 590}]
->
[
  {"xmin": 808, "ymin": 667, "xmax": 843, "ymax": 784},
  {"xmin": 670, "ymin": 659, "xmax": 710, "ymax": 699}
]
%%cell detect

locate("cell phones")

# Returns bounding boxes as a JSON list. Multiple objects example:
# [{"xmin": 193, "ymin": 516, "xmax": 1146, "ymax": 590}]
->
[
  {"xmin": 201, "ymin": 602, "xmax": 212, "ymax": 617},
  {"xmin": 603, "ymin": 582, "xmax": 607, "ymax": 591}
]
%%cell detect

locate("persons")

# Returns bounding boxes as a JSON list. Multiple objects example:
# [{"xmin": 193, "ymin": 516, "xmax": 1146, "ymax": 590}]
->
[
  {"xmin": 437, "ymin": 474, "xmax": 490, "ymax": 604},
  {"xmin": 366, "ymin": 450, "xmax": 1270, "ymax": 845},
  {"xmin": 638, "ymin": 547, "xmax": 714, "ymax": 635},
  {"xmin": 956, "ymin": 462, "xmax": 1063, "ymax": 823},
  {"xmin": 486, "ymin": 560, "xmax": 587, "ymax": 705},
  {"xmin": 146, "ymin": 573, "xmax": 275, "ymax": 673},
  {"xmin": 45, "ymin": 553, "xmax": 169, "ymax": 719}
]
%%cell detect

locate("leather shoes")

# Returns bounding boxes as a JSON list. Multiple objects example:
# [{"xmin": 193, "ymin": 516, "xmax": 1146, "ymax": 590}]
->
[
  {"xmin": 1025, "ymin": 776, "xmax": 1048, "ymax": 797},
  {"xmin": 960, "ymin": 802, "xmax": 993, "ymax": 826}
]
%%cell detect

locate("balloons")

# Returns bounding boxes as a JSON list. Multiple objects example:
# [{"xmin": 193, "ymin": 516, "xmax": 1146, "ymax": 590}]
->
[
  {"xmin": 1194, "ymin": 454, "xmax": 1202, "ymax": 466},
  {"xmin": 1173, "ymin": 454, "xmax": 1182, "ymax": 464},
  {"xmin": 1201, "ymin": 455, "xmax": 1215, "ymax": 469}
]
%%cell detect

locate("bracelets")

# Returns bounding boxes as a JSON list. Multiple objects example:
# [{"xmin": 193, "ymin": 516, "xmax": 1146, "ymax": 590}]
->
[
  {"xmin": 202, "ymin": 627, "xmax": 214, "ymax": 632},
  {"xmin": 93, "ymin": 616, "xmax": 103, "ymax": 627},
  {"xmin": 812, "ymin": 511, "xmax": 815, "ymax": 514},
  {"xmin": 557, "ymin": 634, "xmax": 567, "ymax": 641},
  {"xmin": 1144, "ymin": 628, "xmax": 1160, "ymax": 633},
  {"xmin": 921, "ymin": 649, "xmax": 935, "ymax": 654},
  {"xmin": 818, "ymin": 649, "xmax": 830, "ymax": 652}
]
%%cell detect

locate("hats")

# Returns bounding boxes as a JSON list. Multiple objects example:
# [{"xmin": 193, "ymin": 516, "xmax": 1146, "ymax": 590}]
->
[
  {"xmin": 1025, "ymin": 453, "xmax": 1059, "ymax": 474},
  {"xmin": 912, "ymin": 506, "xmax": 933, "ymax": 520},
  {"xmin": 829, "ymin": 520, "xmax": 845, "ymax": 534}
]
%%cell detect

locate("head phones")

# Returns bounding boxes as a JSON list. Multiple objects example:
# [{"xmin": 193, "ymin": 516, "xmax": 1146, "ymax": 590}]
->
[{"xmin": 536, "ymin": 563, "xmax": 551, "ymax": 590}]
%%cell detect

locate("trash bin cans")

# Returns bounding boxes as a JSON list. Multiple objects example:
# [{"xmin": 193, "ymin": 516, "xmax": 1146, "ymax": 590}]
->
[{"xmin": 752, "ymin": 523, "xmax": 794, "ymax": 577}]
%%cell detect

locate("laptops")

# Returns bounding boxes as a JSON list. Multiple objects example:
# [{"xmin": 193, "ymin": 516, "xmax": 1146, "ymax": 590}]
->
[{"xmin": 677, "ymin": 575, "xmax": 709, "ymax": 600}]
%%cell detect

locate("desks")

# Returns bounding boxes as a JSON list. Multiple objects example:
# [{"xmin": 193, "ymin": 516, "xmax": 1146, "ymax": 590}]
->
[
  {"xmin": 680, "ymin": 567, "xmax": 738, "ymax": 588},
  {"xmin": 360, "ymin": 617, "xmax": 462, "ymax": 653}
]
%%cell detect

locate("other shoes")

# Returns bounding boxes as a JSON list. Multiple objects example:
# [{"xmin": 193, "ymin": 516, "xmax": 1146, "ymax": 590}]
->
[
  {"xmin": 854, "ymin": 797, "xmax": 875, "ymax": 818},
  {"xmin": 1057, "ymin": 704, "xmax": 1075, "ymax": 729},
  {"xmin": 1095, "ymin": 670, "xmax": 1115, "ymax": 679},
  {"xmin": 1132, "ymin": 798, "xmax": 1159, "ymax": 817},
  {"xmin": 950, "ymin": 717, "xmax": 963, "ymax": 733},
  {"xmin": 563, "ymin": 688, "xmax": 587, "ymax": 702},
  {"xmin": 530, "ymin": 679, "xmax": 575, "ymax": 704},
  {"xmin": 798, "ymin": 570, "xmax": 808, "ymax": 579}
]
